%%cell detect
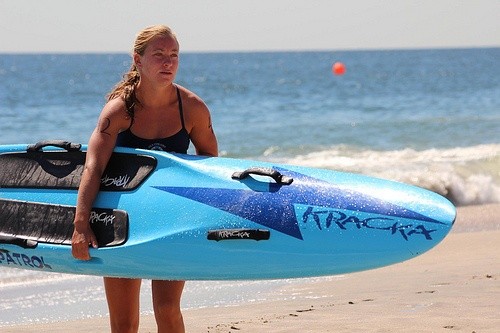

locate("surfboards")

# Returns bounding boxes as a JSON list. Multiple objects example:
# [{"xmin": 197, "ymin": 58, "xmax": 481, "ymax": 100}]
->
[{"xmin": 0, "ymin": 139, "xmax": 459, "ymax": 281}]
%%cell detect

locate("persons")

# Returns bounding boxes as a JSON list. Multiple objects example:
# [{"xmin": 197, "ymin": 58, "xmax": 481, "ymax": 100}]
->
[{"xmin": 71, "ymin": 24, "xmax": 219, "ymax": 333}]
